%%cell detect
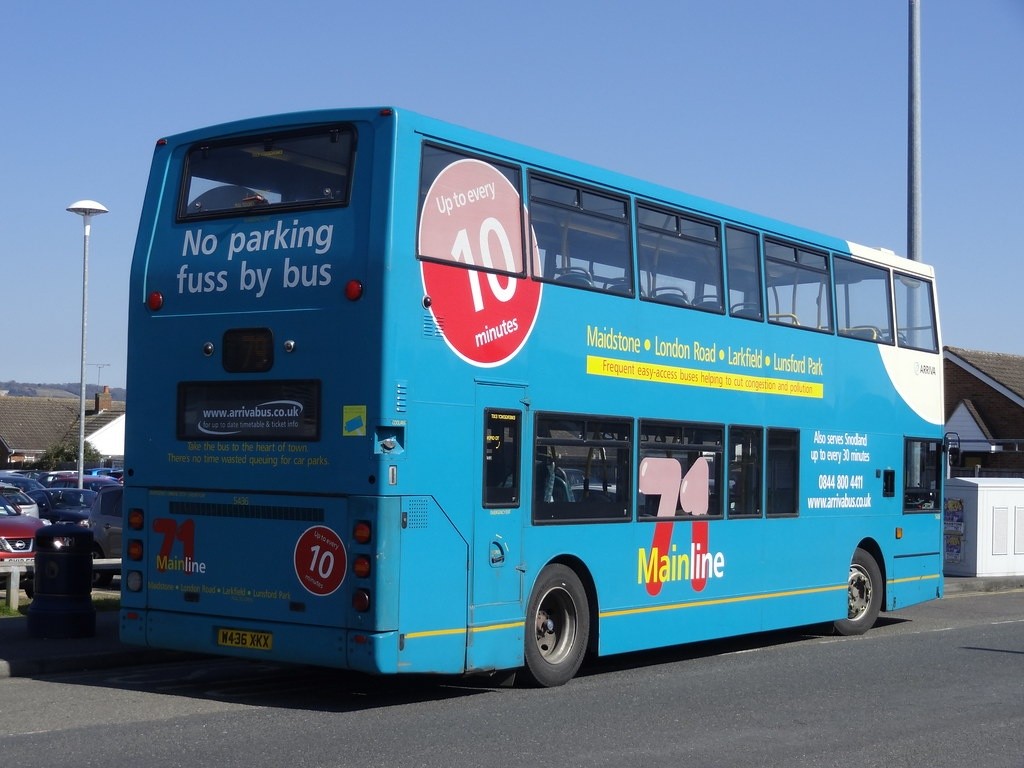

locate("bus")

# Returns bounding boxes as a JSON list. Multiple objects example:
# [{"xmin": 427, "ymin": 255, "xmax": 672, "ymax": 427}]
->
[{"xmin": 120, "ymin": 105, "xmax": 945, "ymax": 687}]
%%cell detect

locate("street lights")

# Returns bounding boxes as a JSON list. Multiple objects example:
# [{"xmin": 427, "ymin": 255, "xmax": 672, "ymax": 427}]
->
[{"xmin": 66, "ymin": 199, "xmax": 106, "ymax": 491}]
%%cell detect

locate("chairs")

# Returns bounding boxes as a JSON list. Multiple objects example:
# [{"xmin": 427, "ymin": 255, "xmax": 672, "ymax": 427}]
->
[
  {"xmin": 551, "ymin": 267, "xmax": 909, "ymax": 349},
  {"xmin": 553, "ymin": 467, "xmax": 576, "ymax": 503}
]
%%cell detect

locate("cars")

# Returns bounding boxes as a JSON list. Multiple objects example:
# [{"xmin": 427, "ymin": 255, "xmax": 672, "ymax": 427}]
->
[{"xmin": 0, "ymin": 465, "xmax": 124, "ymax": 582}]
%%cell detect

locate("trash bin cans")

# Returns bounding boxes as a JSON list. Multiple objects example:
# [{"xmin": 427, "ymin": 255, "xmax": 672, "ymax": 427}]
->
[{"xmin": 27, "ymin": 524, "xmax": 97, "ymax": 636}]
